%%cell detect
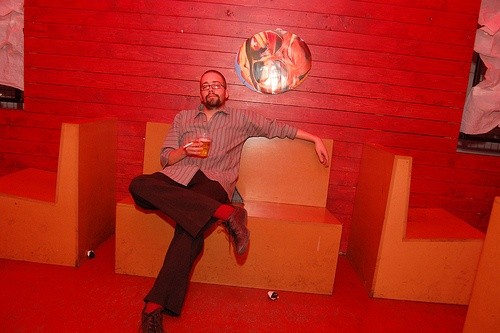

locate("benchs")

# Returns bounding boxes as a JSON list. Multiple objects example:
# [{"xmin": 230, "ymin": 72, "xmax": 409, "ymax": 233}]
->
[
  {"xmin": 463, "ymin": 197, "xmax": 500, "ymax": 333},
  {"xmin": 114, "ymin": 122, "xmax": 342, "ymax": 295},
  {"xmin": 347, "ymin": 142, "xmax": 486, "ymax": 305},
  {"xmin": 0, "ymin": 118, "xmax": 113, "ymax": 267}
]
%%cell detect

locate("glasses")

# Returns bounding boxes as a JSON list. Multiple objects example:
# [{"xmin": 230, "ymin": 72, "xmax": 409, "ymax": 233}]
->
[{"xmin": 200, "ymin": 82, "xmax": 226, "ymax": 91}]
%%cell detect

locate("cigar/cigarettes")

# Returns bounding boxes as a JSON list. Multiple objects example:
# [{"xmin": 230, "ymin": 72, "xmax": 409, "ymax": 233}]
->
[{"xmin": 182, "ymin": 142, "xmax": 194, "ymax": 149}]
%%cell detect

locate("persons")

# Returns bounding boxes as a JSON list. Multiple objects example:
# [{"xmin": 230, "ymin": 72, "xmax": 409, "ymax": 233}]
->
[{"xmin": 129, "ymin": 70, "xmax": 329, "ymax": 333}]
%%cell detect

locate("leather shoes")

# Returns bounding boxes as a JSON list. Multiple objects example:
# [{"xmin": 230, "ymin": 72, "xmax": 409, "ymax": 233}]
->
[
  {"xmin": 222, "ymin": 204, "xmax": 251, "ymax": 254},
  {"xmin": 140, "ymin": 303, "xmax": 164, "ymax": 333}
]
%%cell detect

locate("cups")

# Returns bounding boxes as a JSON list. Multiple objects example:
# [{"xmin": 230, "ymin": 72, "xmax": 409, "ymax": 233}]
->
[{"xmin": 197, "ymin": 133, "xmax": 212, "ymax": 158}]
